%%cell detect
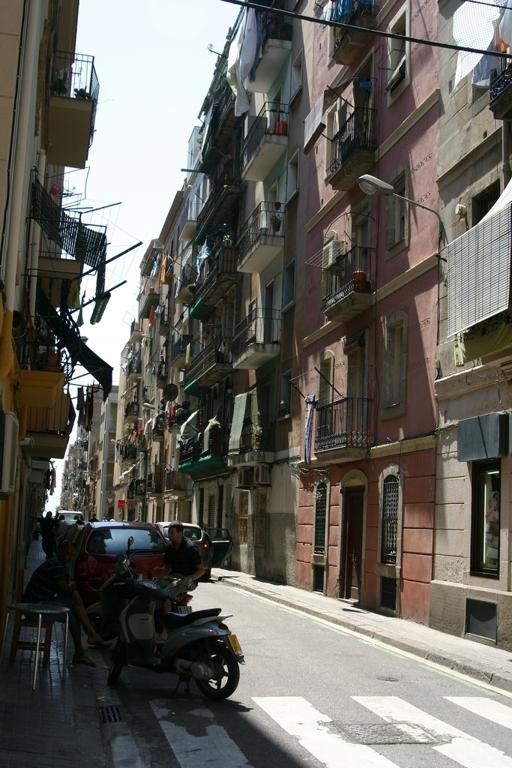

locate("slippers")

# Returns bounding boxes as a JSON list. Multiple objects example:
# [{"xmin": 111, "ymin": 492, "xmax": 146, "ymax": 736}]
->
[
  {"xmin": 73, "ymin": 657, "xmax": 95, "ymax": 667},
  {"xmin": 87, "ymin": 642, "xmax": 111, "ymax": 650}
]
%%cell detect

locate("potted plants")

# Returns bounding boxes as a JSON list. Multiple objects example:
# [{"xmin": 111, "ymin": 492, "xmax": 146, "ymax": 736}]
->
[
  {"xmin": 74, "ymin": 86, "xmax": 92, "ymax": 100},
  {"xmin": 46, "ymin": 70, "xmax": 68, "ymax": 96}
]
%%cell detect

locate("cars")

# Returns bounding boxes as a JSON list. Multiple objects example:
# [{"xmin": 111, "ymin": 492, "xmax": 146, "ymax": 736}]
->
[{"xmin": 54, "ymin": 509, "xmax": 233, "ymax": 644}]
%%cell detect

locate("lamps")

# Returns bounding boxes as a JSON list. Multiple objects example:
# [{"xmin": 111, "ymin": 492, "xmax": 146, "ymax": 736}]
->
[{"xmin": 356, "ymin": 173, "xmax": 443, "ymax": 232}]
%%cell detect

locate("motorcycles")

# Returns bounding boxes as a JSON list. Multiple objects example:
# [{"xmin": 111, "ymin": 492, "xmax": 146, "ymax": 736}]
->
[{"xmin": 107, "ymin": 535, "xmax": 245, "ymax": 702}]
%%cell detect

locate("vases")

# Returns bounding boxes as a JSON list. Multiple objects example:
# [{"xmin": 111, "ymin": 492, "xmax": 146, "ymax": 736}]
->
[
  {"xmin": 352, "ymin": 270, "xmax": 366, "ymax": 289},
  {"xmin": 274, "ymin": 120, "xmax": 288, "ymax": 135}
]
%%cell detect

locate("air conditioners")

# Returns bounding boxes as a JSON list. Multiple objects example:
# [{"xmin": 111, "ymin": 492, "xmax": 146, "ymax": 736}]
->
[{"xmin": 321, "ymin": 240, "xmax": 345, "ymax": 274}]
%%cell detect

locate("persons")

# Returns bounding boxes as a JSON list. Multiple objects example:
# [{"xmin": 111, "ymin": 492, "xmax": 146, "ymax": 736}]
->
[
  {"xmin": 40, "ymin": 511, "xmax": 84, "ymax": 559},
  {"xmin": 20, "ymin": 542, "xmax": 113, "ymax": 667},
  {"xmin": 153, "ymin": 523, "xmax": 206, "ymax": 640},
  {"xmin": 89, "ymin": 513, "xmax": 99, "ymax": 522}
]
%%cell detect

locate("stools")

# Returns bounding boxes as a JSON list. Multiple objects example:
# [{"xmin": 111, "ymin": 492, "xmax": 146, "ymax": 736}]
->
[{"xmin": 1, "ymin": 603, "xmax": 72, "ymax": 692}]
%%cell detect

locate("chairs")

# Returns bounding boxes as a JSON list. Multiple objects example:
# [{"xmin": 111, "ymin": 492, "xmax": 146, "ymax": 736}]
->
[{"xmin": 10, "ymin": 541, "xmax": 53, "ymax": 665}]
